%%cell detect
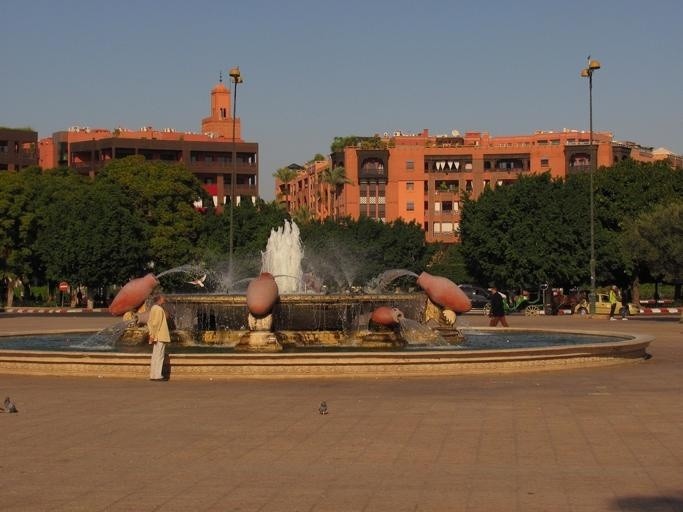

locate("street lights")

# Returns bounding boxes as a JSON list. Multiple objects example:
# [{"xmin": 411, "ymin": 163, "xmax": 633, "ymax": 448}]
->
[
  {"xmin": 578, "ymin": 54, "xmax": 602, "ymax": 316},
  {"xmin": 224, "ymin": 64, "xmax": 245, "ymax": 276}
]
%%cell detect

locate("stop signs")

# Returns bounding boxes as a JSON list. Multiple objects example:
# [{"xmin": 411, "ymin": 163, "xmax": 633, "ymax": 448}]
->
[{"xmin": 59, "ymin": 281, "xmax": 68, "ymax": 292}]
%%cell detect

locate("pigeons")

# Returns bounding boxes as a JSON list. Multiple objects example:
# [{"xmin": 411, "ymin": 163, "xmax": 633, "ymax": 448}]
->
[
  {"xmin": 186, "ymin": 274, "xmax": 207, "ymax": 287},
  {"xmin": 319, "ymin": 401, "xmax": 328, "ymax": 414},
  {"xmin": 4, "ymin": 398, "xmax": 18, "ymax": 412}
]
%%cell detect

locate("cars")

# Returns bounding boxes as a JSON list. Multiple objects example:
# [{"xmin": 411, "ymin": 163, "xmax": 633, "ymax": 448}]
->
[{"xmin": 454, "ymin": 283, "xmax": 645, "ymax": 316}]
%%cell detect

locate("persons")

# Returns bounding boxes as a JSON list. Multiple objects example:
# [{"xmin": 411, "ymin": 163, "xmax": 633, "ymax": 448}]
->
[
  {"xmin": 147, "ymin": 293, "xmax": 171, "ymax": 381},
  {"xmin": 608, "ymin": 285, "xmax": 617, "ymax": 321},
  {"xmin": 489, "ymin": 287, "xmax": 509, "ymax": 327},
  {"xmin": 621, "ymin": 287, "xmax": 629, "ymax": 320},
  {"xmin": 550, "ymin": 291, "xmax": 560, "ymax": 315},
  {"xmin": 13, "ymin": 289, "xmax": 107, "ymax": 304}
]
